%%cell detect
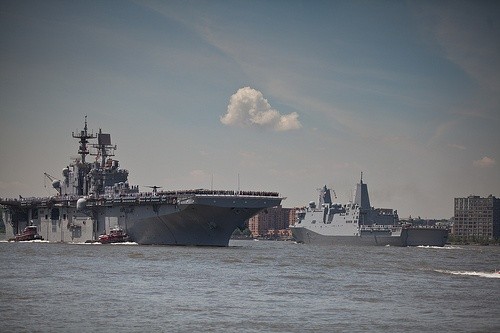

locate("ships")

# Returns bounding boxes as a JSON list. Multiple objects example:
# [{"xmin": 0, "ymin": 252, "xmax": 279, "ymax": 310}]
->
[{"xmin": 0, "ymin": 114, "xmax": 288, "ymax": 246}]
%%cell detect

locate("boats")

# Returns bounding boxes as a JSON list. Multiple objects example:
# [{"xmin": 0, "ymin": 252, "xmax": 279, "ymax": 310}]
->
[
  {"xmin": 289, "ymin": 171, "xmax": 449, "ymax": 249},
  {"xmin": 96, "ymin": 225, "xmax": 128, "ymax": 244},
  {"xmin": 7, "ymin": 225, "xmax": 42, "ymax": 242}
]
